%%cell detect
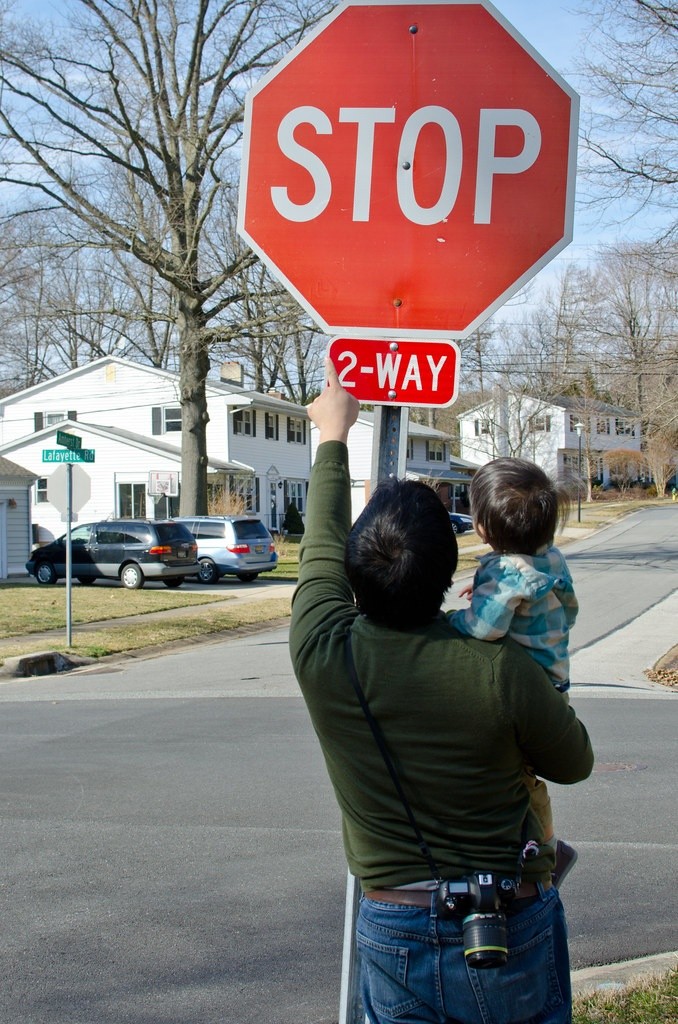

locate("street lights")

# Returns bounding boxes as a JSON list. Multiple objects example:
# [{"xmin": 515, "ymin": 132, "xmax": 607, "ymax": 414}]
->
[{"xmin": 574, "ymin": 422, "xmax": 583, "ymax": 522}]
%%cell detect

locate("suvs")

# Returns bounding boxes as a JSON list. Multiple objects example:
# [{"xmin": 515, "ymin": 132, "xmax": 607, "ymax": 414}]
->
[
  {"xmin": 25, "ymin": 517, "xmax": 201, "ymax": 590},
  {"xmin": 152, "ymin": 515, "xmax": 280, "ymax": 583}
]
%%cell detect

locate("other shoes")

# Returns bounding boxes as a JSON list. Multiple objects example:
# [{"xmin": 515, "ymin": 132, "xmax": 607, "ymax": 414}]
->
[{"xmin": 553, "ymin": 839, "xmax": 578, "ymax": 893}]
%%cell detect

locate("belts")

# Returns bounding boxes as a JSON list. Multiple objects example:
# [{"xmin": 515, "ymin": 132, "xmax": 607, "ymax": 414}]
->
[{"xmin": 365, "ymin": 877, "xmax": 552, "ymax": 911}]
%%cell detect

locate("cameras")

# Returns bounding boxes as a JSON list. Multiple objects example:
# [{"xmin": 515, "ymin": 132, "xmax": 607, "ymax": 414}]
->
[{"xmin": 434, "ymin": 870, "xmax": 519, "ymax": 970}]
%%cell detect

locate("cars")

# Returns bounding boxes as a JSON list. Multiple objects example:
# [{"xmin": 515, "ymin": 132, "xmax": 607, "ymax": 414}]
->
[
  {"xmin": 447, "ymin": 512, "xmax": 474, "ymax": 535},
  {"xmin": 666, "ymin": 475, "xmax": 678, "ymax": 494}
]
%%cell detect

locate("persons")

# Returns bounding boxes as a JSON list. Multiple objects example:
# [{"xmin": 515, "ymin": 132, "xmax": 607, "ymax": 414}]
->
[
  {"xmin": 289, "ymin": 356, "xmax": 594, "ymax": 1024},
  {"xmin": 445, "ymin": 456, "xmax": 579, "ymax": 891}
]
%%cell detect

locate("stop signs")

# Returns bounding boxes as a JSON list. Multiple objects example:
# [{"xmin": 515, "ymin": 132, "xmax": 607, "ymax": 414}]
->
[{"xmin": 233, "ymin": 0, "xmax": 580, "ymax": 342}]
